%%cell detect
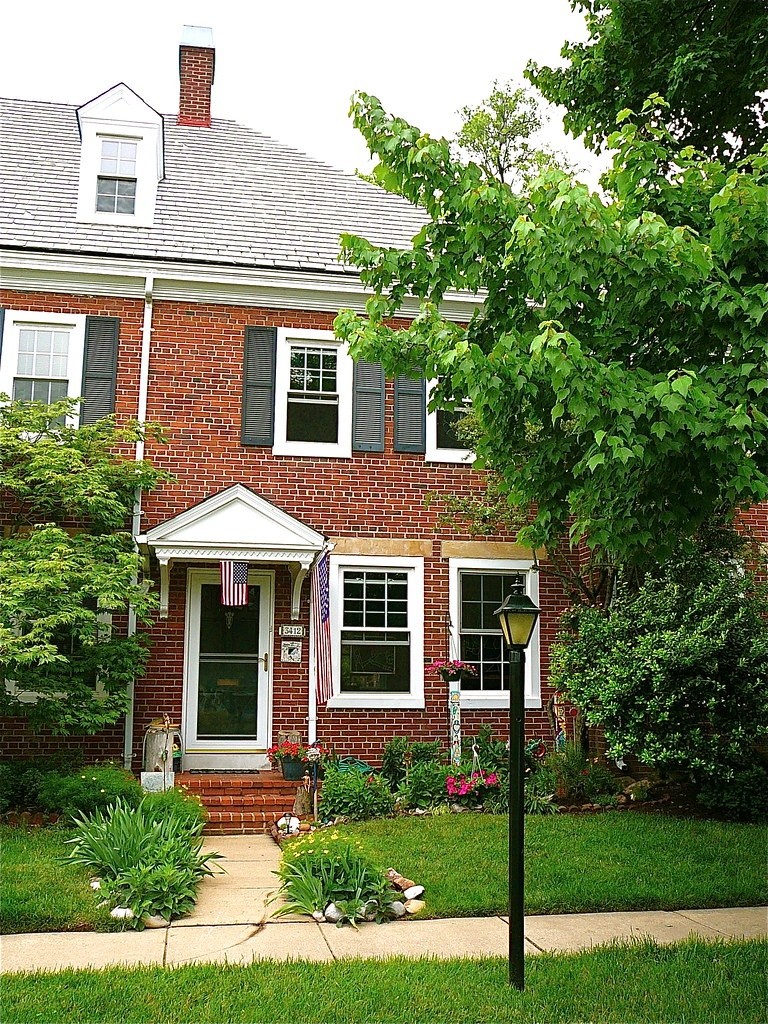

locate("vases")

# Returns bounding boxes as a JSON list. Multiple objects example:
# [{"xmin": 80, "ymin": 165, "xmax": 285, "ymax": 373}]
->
[
  {"xmin": 442, "ymin": 670, "xmax": 462, "ymax": 681},
  {"xmin": 280, "ymin": 756, "xmax": 311, "ymax": 780}
]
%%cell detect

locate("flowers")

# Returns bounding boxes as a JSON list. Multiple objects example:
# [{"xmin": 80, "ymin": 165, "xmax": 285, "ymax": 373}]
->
[
  {"xmin": 266, "ymin": 741, "xmax": 330, "ymax": 763},
  {"xmin": 424, "ymin": 660, "xmax": 479, "ymax": 676}
]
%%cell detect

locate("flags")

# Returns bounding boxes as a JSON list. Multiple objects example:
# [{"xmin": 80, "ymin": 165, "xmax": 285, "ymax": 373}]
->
[
  {"xmin": 220, "ymin": 562, "xmax": 248, "ymax": 606},
  {"xmin": 310, "ymin": 553, "xmax": 334, "ymax": 705}
]
